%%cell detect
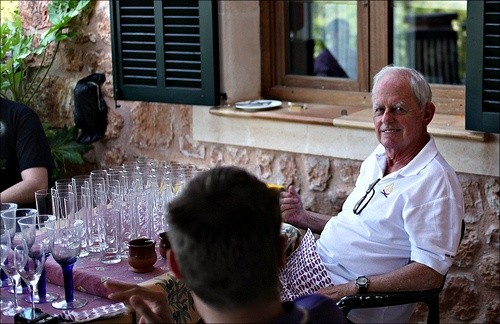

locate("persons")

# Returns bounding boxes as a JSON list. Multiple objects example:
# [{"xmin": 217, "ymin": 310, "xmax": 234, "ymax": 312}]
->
[
  {"xmin": 280, "ymin": 63, "xmax": 464, "ymax": 324},
  {"xmin": 313, "ymin": 18, "xmax": 357, "ymax": 77},
  {"xmin": 105, "ymin": 167, "xmax": 348, "ymax": 323},
  {"xmin": 0, "ymin": 97, "xmax": 51, "ymax": 230}
]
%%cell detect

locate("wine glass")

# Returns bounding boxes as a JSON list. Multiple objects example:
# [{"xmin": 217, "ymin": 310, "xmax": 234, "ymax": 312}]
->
[
  {"xmin": 1, "ymin": 208, "xmax": 38, "ymax": 294},
  {"xmin": 0, "ymin": 229, "xmax": 12, "ymax": 311},
  {"xmin": 17, "ymin": 214, "xmax": 61, "ymax": 302},
  {"xmin": 45, "ymin": 218, "xmax": 88, "ymax": 309},
  {"xmin": 14, "ymin": 243, "xmax": 45, "ymax": 320},
  {"xmin": 0, "ymin": 234, "xmax": 28, "ymax": 317},
  {"xmin": 0, "ymin": 202, "xmax": 17, "ymax": 288}
]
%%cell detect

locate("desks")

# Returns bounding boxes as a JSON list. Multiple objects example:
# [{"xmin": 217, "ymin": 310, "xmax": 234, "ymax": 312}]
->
[
  {"xmin": 1, "ymin": 190, "xmax": 298, "ymax": 324},
  {"xmin": 0, "ymin": 276, "xmax": 133, "ymax": 324}
]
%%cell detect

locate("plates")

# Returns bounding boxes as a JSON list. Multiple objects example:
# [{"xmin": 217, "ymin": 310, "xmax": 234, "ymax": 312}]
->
[{"xmin": 235, "ymin": 99, "xmax": 283, "ymax": 111}]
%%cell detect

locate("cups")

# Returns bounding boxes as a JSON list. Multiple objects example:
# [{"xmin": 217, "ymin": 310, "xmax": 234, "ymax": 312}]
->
[{"xmin": 35, "ymin": 159, "xmax": 211, "ymax": 274}]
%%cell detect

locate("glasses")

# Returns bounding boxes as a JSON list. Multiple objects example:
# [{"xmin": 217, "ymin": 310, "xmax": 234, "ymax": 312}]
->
[{"xmin": 353, "ymin": 179, "xmax": 380, "ymax": 215}]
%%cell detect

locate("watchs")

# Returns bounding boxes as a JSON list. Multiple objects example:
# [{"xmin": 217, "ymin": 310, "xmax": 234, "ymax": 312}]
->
[{"xmin": 355, "ymin": 274, "xmax": 370, "ymax": 293}]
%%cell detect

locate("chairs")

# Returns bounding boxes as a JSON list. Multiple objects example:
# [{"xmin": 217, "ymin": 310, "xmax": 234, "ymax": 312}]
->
[{"xmin": 334, "ymin": 219, "xmax": 465, "ymax": 323}]
